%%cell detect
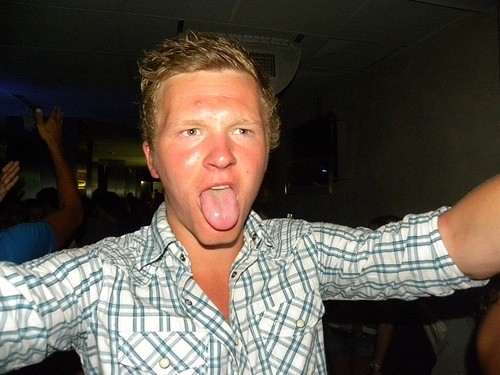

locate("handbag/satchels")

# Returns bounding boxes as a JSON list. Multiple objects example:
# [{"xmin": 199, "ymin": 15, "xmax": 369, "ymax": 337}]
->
[{"xmin": 417, "ymin": 299, "xmax": 449, "ymax": 355}]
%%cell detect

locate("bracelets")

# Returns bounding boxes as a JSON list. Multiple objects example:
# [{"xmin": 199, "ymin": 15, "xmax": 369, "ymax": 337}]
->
[{"xmin": 369, "ymin": 360, "xmax": 385, "ymax": 369}]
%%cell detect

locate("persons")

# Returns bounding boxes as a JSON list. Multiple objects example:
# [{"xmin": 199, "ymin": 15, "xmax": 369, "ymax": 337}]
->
[{"xmin": 0, "ymin": 31, "xmax": 500, "ymax": 375}]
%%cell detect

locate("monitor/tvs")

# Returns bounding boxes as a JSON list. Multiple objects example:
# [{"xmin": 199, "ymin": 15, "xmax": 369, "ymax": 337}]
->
[{"xmin": 292, "ymin": 113, "xmax": 339, "ymax": 187}]
